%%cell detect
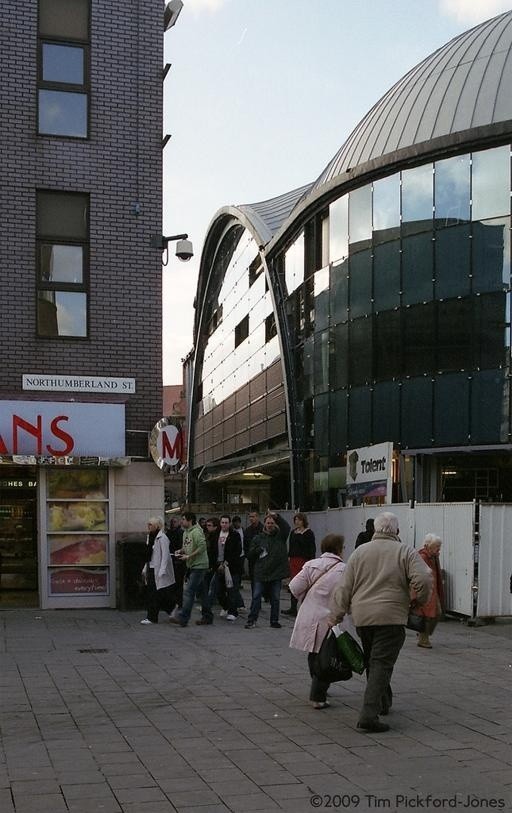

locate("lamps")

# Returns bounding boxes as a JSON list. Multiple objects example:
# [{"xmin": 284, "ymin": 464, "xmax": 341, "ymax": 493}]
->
[{"xmin": 161, "ymin": 233, "xmax": 196, "ymax": 267}]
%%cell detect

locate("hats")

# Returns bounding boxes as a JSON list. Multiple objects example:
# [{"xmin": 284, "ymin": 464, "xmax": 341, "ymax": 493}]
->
[{"xmin": 150, "ymin": 517, "xmax": 164, "ymax": 528}]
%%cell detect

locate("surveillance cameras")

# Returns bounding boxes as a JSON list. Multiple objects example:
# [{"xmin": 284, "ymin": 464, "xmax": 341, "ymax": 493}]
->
[{"xmin": 175, "ymin": 240, "xmax": 194, "ymax": 263}]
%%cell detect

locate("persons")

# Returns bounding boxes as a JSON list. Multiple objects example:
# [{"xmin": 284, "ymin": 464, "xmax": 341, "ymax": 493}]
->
[
  {"xmin": 326, "ymin": 510, "xmax": 435, "ymax": 735},
  {"xmin": 287, "ymin": 533, "xmax": 349, "ymax": 710},
  {"xmin": 127, "ymin": 508, "xmax": 317, "ymax": 630},
  {"xmin": 408, "ymin": 531, "xmax": 444, "ymax": 649},
  {"xmin": 351, "ymin": 517, "xmax": 376, "ymax": 548}
]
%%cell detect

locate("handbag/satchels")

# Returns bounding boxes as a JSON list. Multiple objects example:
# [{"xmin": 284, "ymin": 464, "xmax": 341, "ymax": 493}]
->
[
  {"xmin": 332, "ymin": 626, "xmax": 366, "ymax": 675},
  {"xmin": 318, "ymin": 628, "xmax": 352, "ymax": 681},
  {"xmin": 408, "ymin": 603, "xmax": 427, "ymax": 633}
]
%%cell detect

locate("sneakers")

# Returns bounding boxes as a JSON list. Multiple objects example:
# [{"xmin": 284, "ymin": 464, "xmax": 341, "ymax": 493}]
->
[
  {"xmin": 356, "ymin": 721, "xmax": 389, "ymax": 732},
  {"xmin": 417, "ymin": 640, "xmax": 433, "ymax": 648},
  {"xmin": 313, "ymin": 700, "xmax": 330, "ymax": 709},
  {"xmin": 141, "ymin": 605, "xmax": 297, "ymax": 629}
]
